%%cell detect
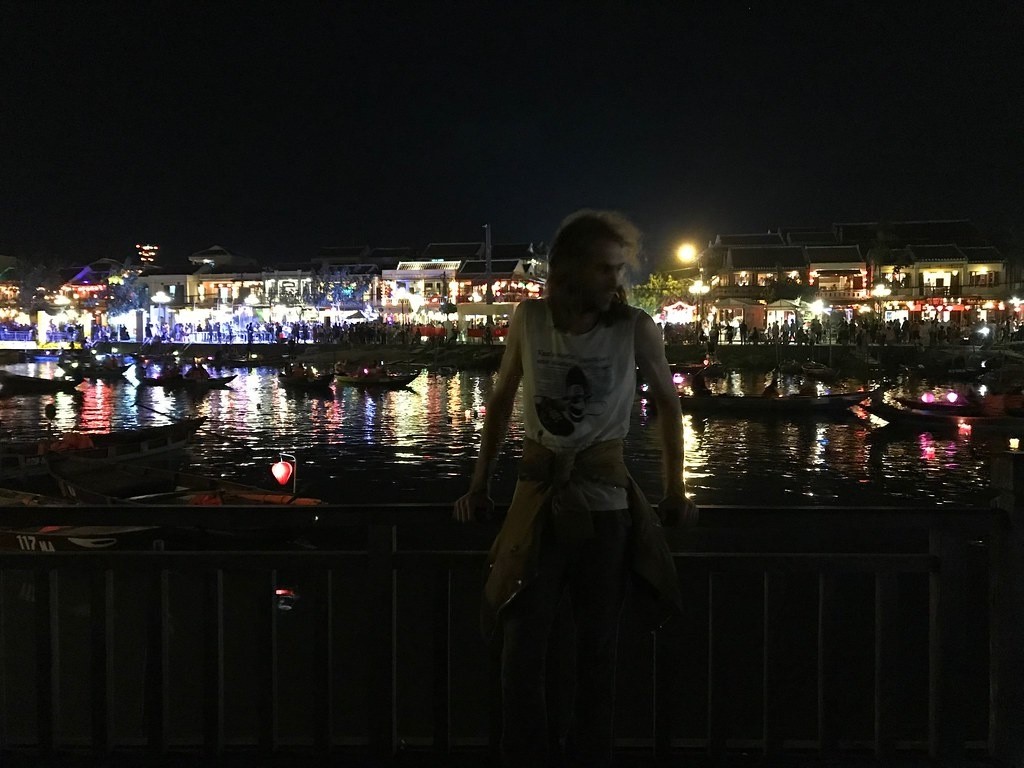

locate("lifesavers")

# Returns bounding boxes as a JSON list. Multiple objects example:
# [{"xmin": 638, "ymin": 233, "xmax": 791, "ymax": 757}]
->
[
  {"xmin": 41, "ymin": 453, "xmax": 76, "ymax": 489},
  {"xmin": 142, "ymin": 457, "xmax": 182, "ymax": 493}
]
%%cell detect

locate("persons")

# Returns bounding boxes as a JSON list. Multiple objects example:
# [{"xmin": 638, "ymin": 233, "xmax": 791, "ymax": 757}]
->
[
  {"xmin": 0, "ymin": 299, "xmax": 1021, "ymax": 353},
  {"xmin": 450, "ymin": 209, "xmax": 699, "ymax": 768}
]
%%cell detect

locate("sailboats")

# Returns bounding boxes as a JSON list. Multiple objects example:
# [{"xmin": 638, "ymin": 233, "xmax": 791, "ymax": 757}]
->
[{"xmin": 667, "ymin": 328, "xmax": 1024, "ymax": 379}]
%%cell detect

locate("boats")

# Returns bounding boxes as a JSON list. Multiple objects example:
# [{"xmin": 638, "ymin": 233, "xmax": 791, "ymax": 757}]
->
[
  {"xmin": 645, "ymin": 390, "xmax": 878, "ymax": 414},
  {"xmin": 0, "ymin": 487, "xmax": 227, "ymax": 552},
  {"xmin": 854, "ymin": 397, "xmax": 1024, "ymax": 431},
  {"xmin": 0, "ymin": 416, "xmax": 208, "ymax": 503},
  {"xmin": 0, "ymin": 345, "xmax": 507, "ymax": 392}
]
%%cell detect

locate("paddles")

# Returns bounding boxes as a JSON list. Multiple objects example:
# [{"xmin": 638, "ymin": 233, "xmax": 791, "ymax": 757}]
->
[{"xmin": 132, "ymin": 398, "xmax": 253, "ymax": 450}]
